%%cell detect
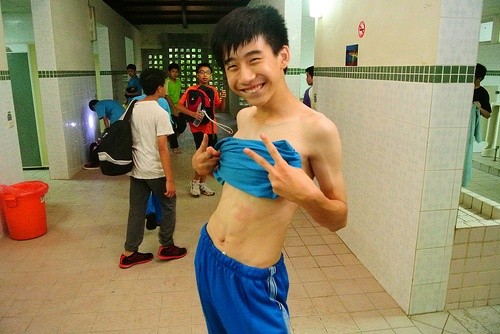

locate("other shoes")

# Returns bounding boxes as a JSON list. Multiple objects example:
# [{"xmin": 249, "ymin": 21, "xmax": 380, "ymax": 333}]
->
[{"xmin": 171, "ymin": 148, "xmax": 184, "ymax": 155}]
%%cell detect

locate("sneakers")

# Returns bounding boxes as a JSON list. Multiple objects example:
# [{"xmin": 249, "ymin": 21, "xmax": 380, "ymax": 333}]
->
[
  {"xmin": 199, "ymin": 182, "xmax": 215, "ymax": 196},
  {"xmin": 83, "ymin": 163, "xmax": 101, "ymax": 170},
  {"xmin": 190, "ymin": 178, "xmax": 201, "ymax": 196},
  {"xmin": 157, "ymin": 245, "xmax": 187, "ymax": 259},
  {"xmin": 146, "ymin": 212, "xmax": 156, "ymax": 230},
  {"xmin": 119, "ymin": 253, "xmax": 154, "ymax": 267}
]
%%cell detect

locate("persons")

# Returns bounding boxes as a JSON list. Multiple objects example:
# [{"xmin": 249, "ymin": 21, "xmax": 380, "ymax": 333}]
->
[
  {"xmin": 301, "ymin": 65, "xmax": 314, "ymax": 109},
  {"xmin": 177, "ymin": 63, "xmax": 226, "ymax": 198},
  {"xmin": 125, "ymin": 65, "xmax": 141, "ymax": 105},
  {"xmin": 165, "ymin": 63, "xmax": 184, "ymax": 154},
  {"xmin": 82, "ymin": 98, "xmax": 126, "ymax": 170},
  {"xmin": 127, "ymin": 95, "xmax": 173, "ymax": 230},
  {"xmin": 117, "ymin": 69, "xmax": 187, "ymax": 269},
  {"xmin": 192, "ymin": 5, "xmax": 348, "ymax": 333},
  {"xmin": 471, "ymin": 62, "xmax": 494, "ymax": 120}
]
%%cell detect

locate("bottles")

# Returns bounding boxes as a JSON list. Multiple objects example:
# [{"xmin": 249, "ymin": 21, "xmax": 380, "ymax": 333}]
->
[{"xmin": 192, "ymin": 109, "xmax": 206, "ymax": 127}]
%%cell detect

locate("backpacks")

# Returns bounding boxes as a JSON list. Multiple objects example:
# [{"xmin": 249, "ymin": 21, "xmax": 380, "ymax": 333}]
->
[{"xmin": 97, "ymin": 99, "xmax": 139, "ymax": 176}]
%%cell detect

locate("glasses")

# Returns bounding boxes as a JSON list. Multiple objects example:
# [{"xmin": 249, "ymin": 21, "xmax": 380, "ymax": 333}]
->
[{"xmin": 198, "ymin": 70, "xmax": 211, "ymax": 75}]
